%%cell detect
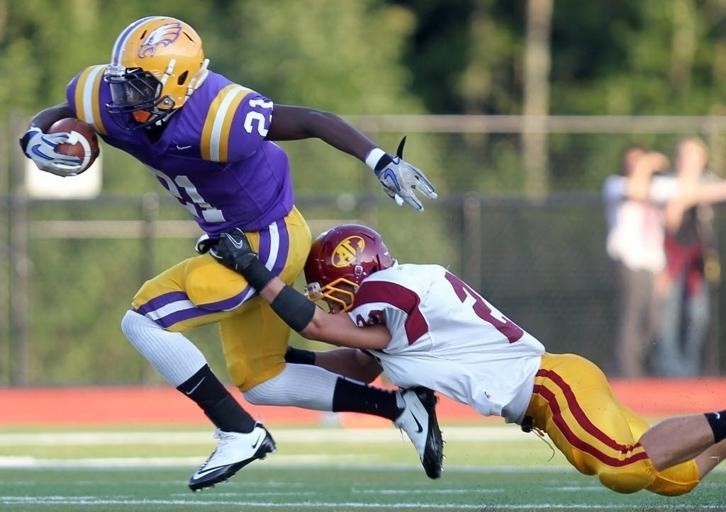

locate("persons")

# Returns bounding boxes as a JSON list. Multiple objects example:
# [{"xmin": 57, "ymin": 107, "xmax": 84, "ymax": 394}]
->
[
  {"xmin": 21, "ymin": 13, "xmax": 447, "ymax": 484},
  {"xmin": 656, "ymin": 132, "xmax": 725, "ymax": 385},
  {"xmin": 599, "ymin": 148, "xmax": 675, "ymax": 381},
  {"xmin": 193, "ymin": 223, "xmax": 726, "ymax": 496}
]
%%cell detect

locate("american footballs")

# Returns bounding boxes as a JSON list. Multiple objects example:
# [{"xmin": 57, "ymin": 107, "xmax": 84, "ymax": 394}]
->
[{"xmin": 48, "ymin": 118, "xmax": 97, "ymax": 174}]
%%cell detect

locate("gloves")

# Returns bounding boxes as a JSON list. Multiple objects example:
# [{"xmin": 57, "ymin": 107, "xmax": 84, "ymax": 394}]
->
[
  {"xmin": 18, "ymin": 126, "xmax": 82, "ymax": 178},
  {"xmin": 373, "ymin": 152, "xmax": 438, "ymax": 213},
  {"xmin": 209, "ymin": 226, "xmax": 257, "ymax": 272}
]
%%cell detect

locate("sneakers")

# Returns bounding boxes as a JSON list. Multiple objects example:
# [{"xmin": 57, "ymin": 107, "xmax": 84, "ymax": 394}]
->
[
  {"xmin": 188, "ymin": 420, "xmax": 277, "ymax": 493},
  {"xmin": 393, "ymin": 385, "xmax": 444, "ymax": 479}
]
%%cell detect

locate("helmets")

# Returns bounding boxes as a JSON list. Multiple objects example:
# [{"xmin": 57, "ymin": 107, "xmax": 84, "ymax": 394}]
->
[
  {"xmin": 304, "ymin": 222, "xmax": 395, "ymax": 315},
  {"xmin": 103, "ymin": 14, "xmax": 210, "ymax": 125}
]
